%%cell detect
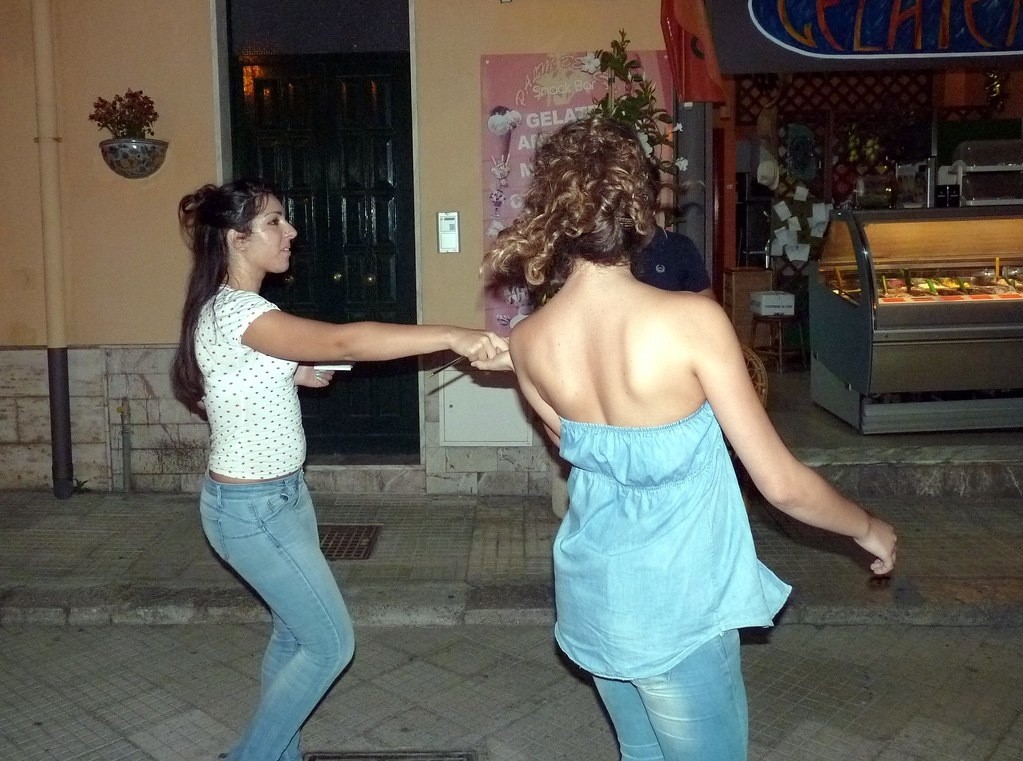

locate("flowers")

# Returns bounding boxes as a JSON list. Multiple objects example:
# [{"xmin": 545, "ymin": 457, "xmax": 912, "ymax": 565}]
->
[{"xmin": 86, "ymin": 85, "xmax": 159, "ymax": 140}]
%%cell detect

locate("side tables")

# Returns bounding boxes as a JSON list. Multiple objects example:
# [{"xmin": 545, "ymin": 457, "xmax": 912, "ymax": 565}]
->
[{"xmin": 749, "ymin": 314, "xmax": 809, "ymax": 374}]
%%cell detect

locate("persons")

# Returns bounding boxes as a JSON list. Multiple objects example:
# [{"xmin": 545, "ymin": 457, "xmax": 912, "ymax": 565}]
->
[
  {"xmin": 629, "ymin": 163, "xmax": 714, "ymax": 299},
  {"xmin": 172, "ymin": 178, "xmax": 510, "ymax": 761},
  {"xmin": 474, "ymin": 120, "xmax": 897, "ymax": 761}
]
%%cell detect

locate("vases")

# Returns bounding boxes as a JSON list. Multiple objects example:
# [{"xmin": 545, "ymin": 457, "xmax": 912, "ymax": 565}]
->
[{"xmin": 98, "ymin": 137, "xmax": 169, "ymax": 179}]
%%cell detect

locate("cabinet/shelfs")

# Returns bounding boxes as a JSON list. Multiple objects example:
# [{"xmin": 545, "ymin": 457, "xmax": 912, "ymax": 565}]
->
[{"xmin": 807, "ymin": 203, "xmax": 1023, "ymax": 434}]
